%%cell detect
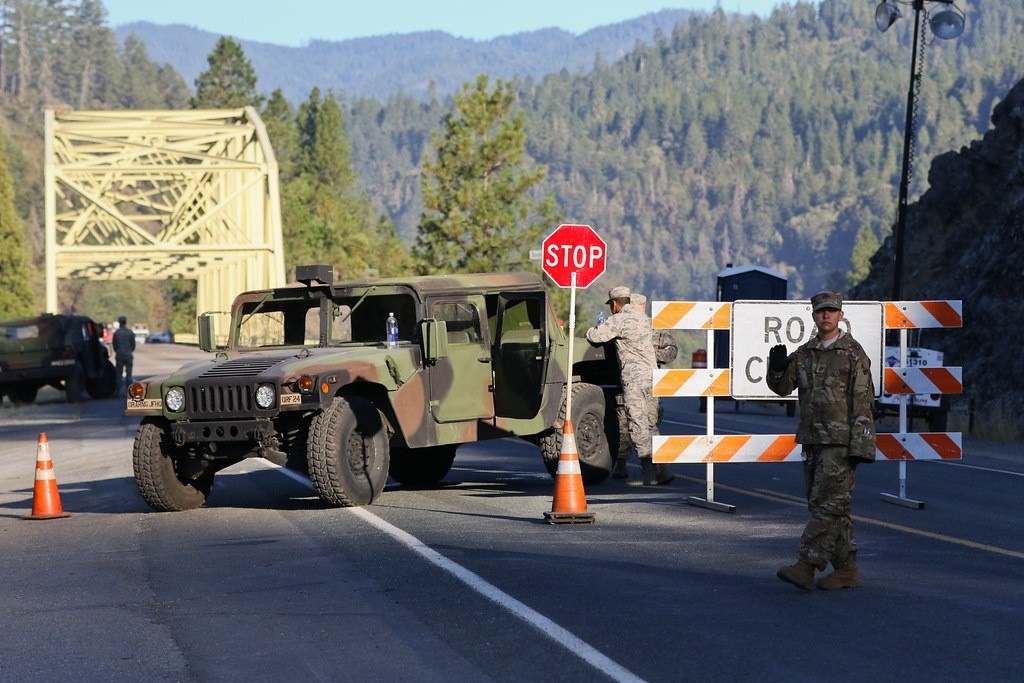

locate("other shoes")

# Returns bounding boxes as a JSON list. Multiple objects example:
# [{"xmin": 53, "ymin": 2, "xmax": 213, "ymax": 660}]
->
[
  {"xmin": 657, "ymin": 463, "xmax": 675, "ymax": 485},
  {"xmin": 816, "ymin": 562, "xmax": 859, "ymax": 589},
  {"xmin": 111, "ymin": 386, "xmax": 120, "ymax": 397},
  {"xmin": 625, "ymin": 460, "xmax": 658, "ymax": 487},
  {"xmin": 612, "ymin": 459, "xmax": 629, "ymax": 479},
  {"xmin": 777, "ymin": 560, "xmax": 815, "ymax": 591}
]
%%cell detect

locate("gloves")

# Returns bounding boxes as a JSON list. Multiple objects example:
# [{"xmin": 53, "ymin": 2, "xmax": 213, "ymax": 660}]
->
[
  {"xmin": 769, "ymin": 344, "xmax": 794, "ymax": 372},
  {"xmin": 850, "ymin": 456, "xmax": 865, "ymax": 471}
]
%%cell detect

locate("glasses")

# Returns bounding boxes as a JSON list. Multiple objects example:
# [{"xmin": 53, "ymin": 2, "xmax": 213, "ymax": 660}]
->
[{"xmin": 815, "ymin": 307, "xmax": 840, "ymax": 313}]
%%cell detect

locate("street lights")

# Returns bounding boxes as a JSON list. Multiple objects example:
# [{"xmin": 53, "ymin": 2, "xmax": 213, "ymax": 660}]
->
[{"xmin": 872, "ymin": 0, "xmax": 967, "ymax": 347}]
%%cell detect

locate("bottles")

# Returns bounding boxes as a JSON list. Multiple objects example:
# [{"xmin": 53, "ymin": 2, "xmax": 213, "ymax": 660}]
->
[
  {"xmin": 597, "ymin": 312, "xmax": 605, "ymax": 323},
  {"xmin": 386, "ymin": 313, "xmax": 399, "ymax": 350}
]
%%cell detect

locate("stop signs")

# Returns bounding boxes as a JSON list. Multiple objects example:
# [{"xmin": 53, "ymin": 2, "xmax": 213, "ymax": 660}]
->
[{"xmin": 540, "ymin": 223, "xmax": 608, "ymax": 291}]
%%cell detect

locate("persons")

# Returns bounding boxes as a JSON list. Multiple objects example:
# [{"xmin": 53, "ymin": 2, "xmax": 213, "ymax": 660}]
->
[
  {"xmin": 586, "ymin": 285, "xmax": 676, "ymax": 487},
  {"xmin": 112, "ymin": 316, "xmax": 136, "ymax": 398},
  {"xmin": 766, "ymin": 292, "xmax": 875, "ymax": 592},
  {"xmin": 609, "ymin": 293, "xmax": 678, "ymax": 480}
]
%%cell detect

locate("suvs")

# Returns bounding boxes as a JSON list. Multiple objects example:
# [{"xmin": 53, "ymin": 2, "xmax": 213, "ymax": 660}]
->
[
  {"xmin": 123, "ymin": 263, "xmax": 603, "ymax": 511},
  {"xmin": 0, "ymin": 311, "xmax": 116, "ymax": 404}
]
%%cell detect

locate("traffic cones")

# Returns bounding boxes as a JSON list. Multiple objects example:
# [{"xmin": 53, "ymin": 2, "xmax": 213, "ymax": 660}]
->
[
  {"xmin": 22, "ymin": 432, "xmax": 72, "ymax": 520},
  {"xmin": 544, "ymin": 419, "xmax": 595, "ymax": 524}
]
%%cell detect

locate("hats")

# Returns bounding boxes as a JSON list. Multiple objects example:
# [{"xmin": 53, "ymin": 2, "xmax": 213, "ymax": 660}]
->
[
  {"xmin": 605, "ymin": 286, "xmax": 630, "ymax": 304},
  {"xmin": 810, "ymin": 291, "xmax": 842, "ymax": 311},
  {"xmin": 630, "ymin": 294, "xmax": 647, "ymax": 305},
  {"xmin": 118, "ymin": 317, "xmax": 126, "ymax": 322}
]
